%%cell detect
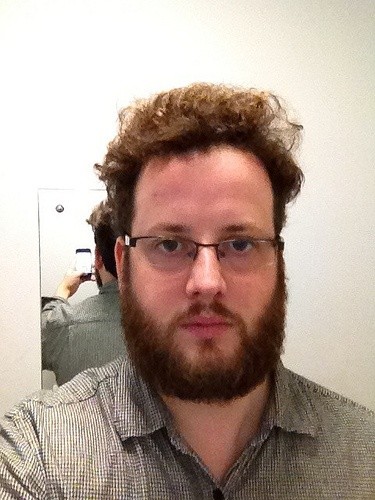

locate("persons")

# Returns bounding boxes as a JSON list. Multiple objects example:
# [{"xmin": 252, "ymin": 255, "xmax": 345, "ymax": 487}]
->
[
  {"xmin": 40, "ymin": 200, "xmax": 128, "ymax": 388},
  {"xmin": 2, "ymin": 82, "xmax": 375, "ymax": 499}
]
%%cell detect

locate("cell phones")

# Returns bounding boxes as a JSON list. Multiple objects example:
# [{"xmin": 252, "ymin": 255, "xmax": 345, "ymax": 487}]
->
[{"xmin": 76, "ymin": 249, "xmax": 92, "ymax": 280}]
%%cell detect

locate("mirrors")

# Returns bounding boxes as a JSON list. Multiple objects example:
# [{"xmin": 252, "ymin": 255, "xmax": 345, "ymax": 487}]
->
[{"xmin": 38, "ymin": 188, "xmax": 128, "ymax": 391}]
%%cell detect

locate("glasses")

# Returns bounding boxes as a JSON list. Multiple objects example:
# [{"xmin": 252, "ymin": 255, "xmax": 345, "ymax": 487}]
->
[{"xmin": 123, "ymin": 232, "xmax": 284, "ymax": 270}]
